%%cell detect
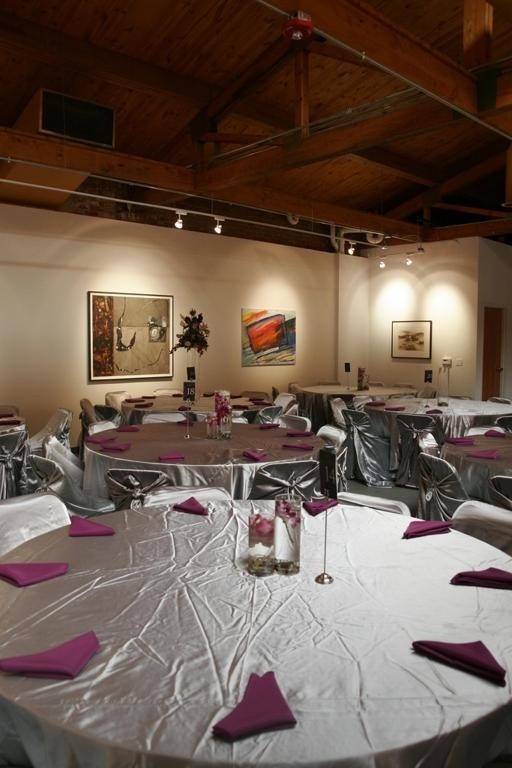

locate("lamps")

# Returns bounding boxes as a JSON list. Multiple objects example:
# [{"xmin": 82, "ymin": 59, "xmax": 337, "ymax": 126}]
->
[
  {"xmin": 348, "ymin": 241, "xmax": 357, "ymax": 256},
  {"xmin": 402, "ymin": 256, "xmax": 413, "ymax": 269},
  {"xmin": 378, "ymin": 258, "xmax": 388, "ymax": 270},
  {"xmin": 214, "ymin": 217, "xmax": 225, "ymax": 234},
  {"xmin": 173, "ymin": 210, "xmax": 187, "ymax": 229}
]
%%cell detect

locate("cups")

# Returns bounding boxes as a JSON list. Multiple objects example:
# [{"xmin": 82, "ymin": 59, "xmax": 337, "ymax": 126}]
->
[
  {"xmin": 206, "ymin": 414, "xmax": 219, "ymax": 439},
  {"xmin": 357, "ymin": 367, "xmax": 369, "ymax": 390},
  {"xmin": 248, "ymin": 513, "xmax": 276, "ymax": 577},
  {"xmin": 276, "ymin": 494, "xmax": 301, "ymax": 575},
  {"xmin": 218, "ymin": 404, "xmax": 233, "ymax": 439}
]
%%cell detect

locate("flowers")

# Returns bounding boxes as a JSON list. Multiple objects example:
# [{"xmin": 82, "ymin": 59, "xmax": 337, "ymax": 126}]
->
[{"xmin": 169, "ymin": 308, "xmax": 211, "ymax": 355}]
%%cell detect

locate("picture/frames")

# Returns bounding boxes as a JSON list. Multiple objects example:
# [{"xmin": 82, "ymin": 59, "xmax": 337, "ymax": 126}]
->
[
  {"xmin": 87, "ymin": 291, "xmax": 174, "ymax": 381},
  {"xmin": 391, "ymin": 320, "xmax": 432, "ymax": 360}
]
